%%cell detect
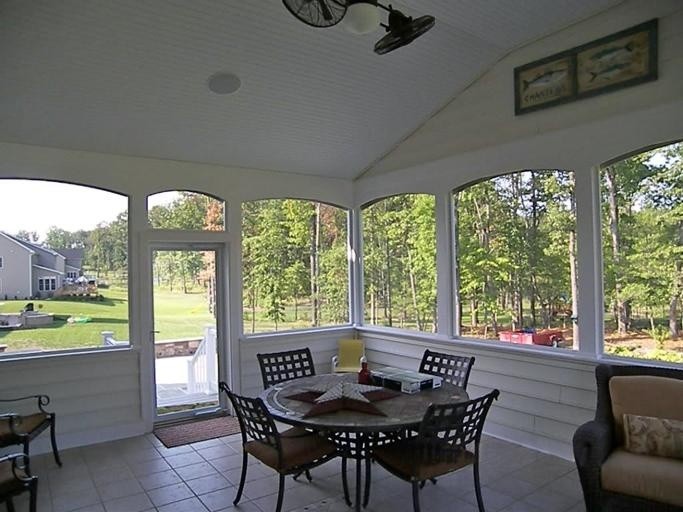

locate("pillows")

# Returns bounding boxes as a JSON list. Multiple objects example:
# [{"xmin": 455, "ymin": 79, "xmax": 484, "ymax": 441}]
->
[{"xmin": 609, "ymin": 374, "xmax": 683, "ymax": 459}]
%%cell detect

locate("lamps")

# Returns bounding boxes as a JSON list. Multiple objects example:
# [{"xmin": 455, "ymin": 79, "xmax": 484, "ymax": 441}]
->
[{"xmin": 344, "ymin": 0, "xmax": 380, "ymax": 36}]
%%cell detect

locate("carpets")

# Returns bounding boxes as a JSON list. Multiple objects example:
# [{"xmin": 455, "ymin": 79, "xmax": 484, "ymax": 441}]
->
[{"xmin": 153, "ymin": 415, "xmax": 242, "ymax": 448}]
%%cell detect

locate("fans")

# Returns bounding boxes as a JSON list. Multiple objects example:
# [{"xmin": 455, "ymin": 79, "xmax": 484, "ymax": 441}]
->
[
  {"xmin": 347, "ymin": 0, "xmax": 436, "ymax": 55},
  {"xmin": 281, "ymin": 0, "xmax": 347, "ymax": 28}
]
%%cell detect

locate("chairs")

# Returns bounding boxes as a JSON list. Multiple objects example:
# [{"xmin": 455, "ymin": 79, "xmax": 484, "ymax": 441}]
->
[
  {"xmin": 572, "ymin": 364, "xmax": 683, "ymax": 512},
  {"xmin": 0, "ymin": 453, "xmax": 39, "ymax": 512},
  {"xmin": 332, "ymin": 339, "xmax": 365, "ymax": 373},
  {"xmin": 0, "ymin": 394, "xmax": 63, "ymax": 477}
]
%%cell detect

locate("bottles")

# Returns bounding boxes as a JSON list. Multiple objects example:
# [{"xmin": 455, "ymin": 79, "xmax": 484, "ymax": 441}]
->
[{"xmin": 357, "ymin": 362, "xmax": 369, "ymax": 384}]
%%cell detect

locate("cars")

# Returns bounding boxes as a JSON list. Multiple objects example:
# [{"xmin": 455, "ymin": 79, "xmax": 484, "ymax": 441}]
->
[{"xmin": 496, "ymin": 326, "xmax": 564, "ymax": 348}]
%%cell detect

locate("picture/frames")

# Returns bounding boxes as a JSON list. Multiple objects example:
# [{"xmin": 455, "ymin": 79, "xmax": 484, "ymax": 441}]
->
[{"xmin": 513, "ymin": 18, "xmax": 658, "ymax": 115}]
